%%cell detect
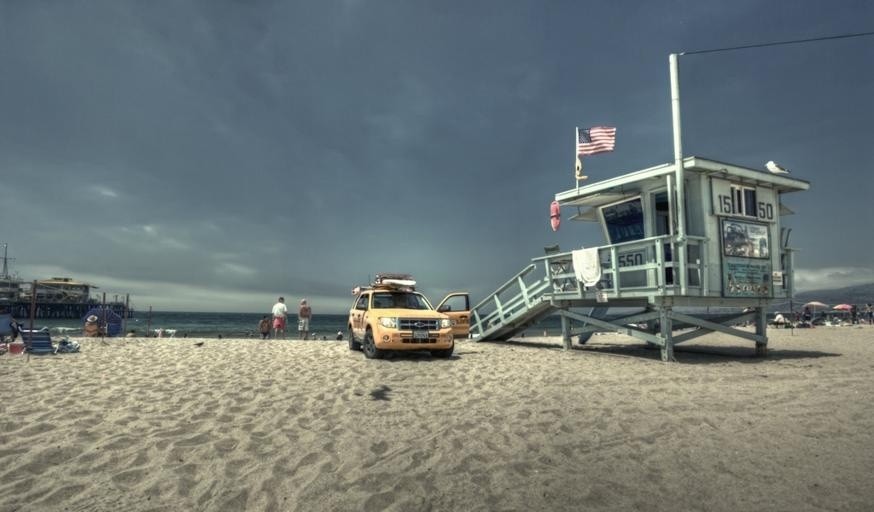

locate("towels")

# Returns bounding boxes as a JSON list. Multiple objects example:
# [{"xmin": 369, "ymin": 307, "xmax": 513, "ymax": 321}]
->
[{"xmin": 571, "ymin": 246, "xmax": 602, "ymax": 287}]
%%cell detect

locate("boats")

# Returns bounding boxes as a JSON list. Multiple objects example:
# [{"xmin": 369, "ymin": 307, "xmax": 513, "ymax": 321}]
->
[
  {"xmin": 0, "ymin": 315, "xmax": 21, "ymax": 342},
  {"xmin": 81, "ymin": 309, "xmax": 122, "ymax": 337}
]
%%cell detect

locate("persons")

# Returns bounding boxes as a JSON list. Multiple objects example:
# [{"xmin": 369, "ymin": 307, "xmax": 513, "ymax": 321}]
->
[
  {"xmin": 126, "ymin": 330, "xmax": 136, "ymax": 337},
  {"xmin": 336, "ymin": 328, "xmax": 343, "ymax": 340},
  {"xmin": 803, "ymin": 304, "xmax": 874, "ymax": 325},
  {"xmin": 85, "ymin": 315, "xmax": 98, "ymax": 337},
  {"xmin": 297, "ymin": 300, "xmax": 312, "ymax": 339},
  {"xmin": 258, "ymin": 315, "xmax": 271, "ymax": 339},
  {"xmin": 770, "ymin": 311, "xmax": 784, "ymax": 328},
  {"xmin": 272, "ymin": 297, "xmax": 288, "ymax": 339}
]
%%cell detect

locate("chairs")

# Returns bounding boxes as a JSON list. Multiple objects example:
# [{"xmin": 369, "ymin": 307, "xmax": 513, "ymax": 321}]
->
[
  {"xmin": 0, "ymin": 313, "xmax": 15, "ymax": 347},
  {"xmin": 545, "ymin": 243, "xmax": 577, "ymax": 291},
  {"xmin": 768, "ymin": 317, "xmax": 855, "ymax": 329},
  {"xmin": 18, "ymin": 325, "xmax": 59, "ymax": 363},
  {"xmin": 82, "ymin": 323, "xmax": 97, "ymax": 337}
]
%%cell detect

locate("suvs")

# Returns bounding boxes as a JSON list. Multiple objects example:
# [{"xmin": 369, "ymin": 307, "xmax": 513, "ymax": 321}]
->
[{"xmin": 347, "ymin": 274, "xmax": 472, "ymax": 358}]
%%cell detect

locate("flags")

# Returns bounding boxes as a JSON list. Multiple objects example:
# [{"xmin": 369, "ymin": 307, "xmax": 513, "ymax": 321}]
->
[{"xmin": 575, "ymin": 125, "xmax": 616, "ymax": 154}]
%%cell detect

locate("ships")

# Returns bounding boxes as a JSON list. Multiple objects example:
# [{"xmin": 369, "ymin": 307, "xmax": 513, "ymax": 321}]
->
[{"xmin": 0, "ymin": 242, "xmax": 147, "ymax": 336}]
